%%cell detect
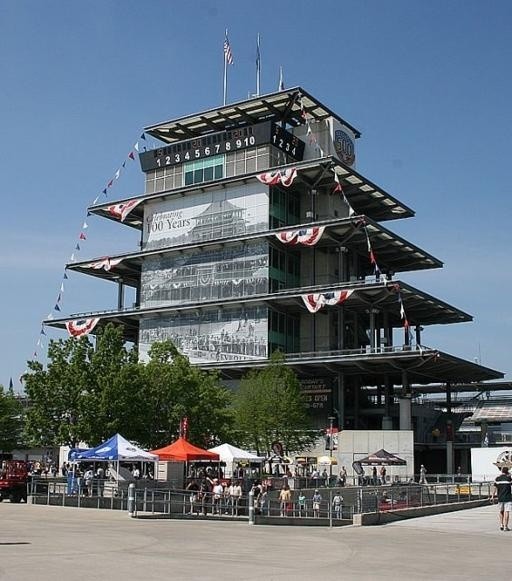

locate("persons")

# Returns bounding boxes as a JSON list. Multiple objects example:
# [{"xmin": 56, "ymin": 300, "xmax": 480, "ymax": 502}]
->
[
  {"xmin": 418, "ymin": 465, "xmax": 428, "ymax": 485},
  {"xmin": 490, "ymin": 467, "xmax": 512, "ymax": 532},
  {"xmin": 371, "ymin": 467, "xmax": 377, "ymax": 486},
  {"xmin": 381, "ymin": 467, "xmax": 386, "ymax": 485}
]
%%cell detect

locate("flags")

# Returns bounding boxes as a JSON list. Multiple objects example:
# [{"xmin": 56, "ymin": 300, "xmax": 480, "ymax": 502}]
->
[{"xmin": 223, "ymin": 35, "xmax": 234, "ymax": 65}]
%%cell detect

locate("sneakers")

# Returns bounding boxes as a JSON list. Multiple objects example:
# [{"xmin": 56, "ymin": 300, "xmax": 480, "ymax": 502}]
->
[{"xmin": 500, "ymin": 524, "xmax": 512, "ymax": 531}]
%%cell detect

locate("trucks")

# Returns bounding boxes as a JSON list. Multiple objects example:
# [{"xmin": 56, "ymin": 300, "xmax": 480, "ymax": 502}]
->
[{"xmin": 0, "ymin": 460, "xmax": 31, "ymax": 502}]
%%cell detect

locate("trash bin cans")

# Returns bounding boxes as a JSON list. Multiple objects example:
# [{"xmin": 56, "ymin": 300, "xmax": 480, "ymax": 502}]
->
[{"xmin": 27, "ymin": 476, "xmax": 40, "ymax": 496}]
